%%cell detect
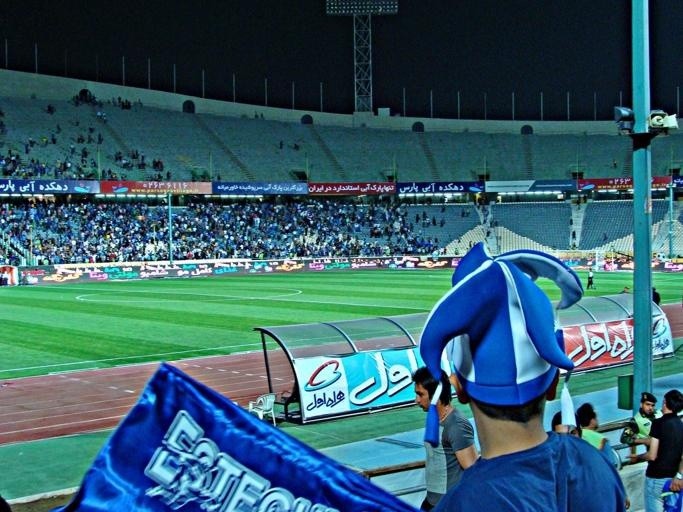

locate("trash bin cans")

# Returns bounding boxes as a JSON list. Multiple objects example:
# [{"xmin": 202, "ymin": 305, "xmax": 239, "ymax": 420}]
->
[{"xmin": 618, "ymin": 374, "xmax": 633, "ymax": 410}]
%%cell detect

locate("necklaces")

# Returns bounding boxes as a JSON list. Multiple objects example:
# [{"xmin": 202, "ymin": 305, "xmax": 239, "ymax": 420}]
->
[{"xmin": 439, "ymin": 404, "xmax": 449, "ymax": 420}]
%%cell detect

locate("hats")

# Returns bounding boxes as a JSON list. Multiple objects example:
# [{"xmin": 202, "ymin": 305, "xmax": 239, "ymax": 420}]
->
[
  {"xmin": 641, "ymin": 392, "xmax": 657, "ymax": 403},
  {"xmin": 419, "ymin": 243, "xmax": 584, "ymax": 405}
]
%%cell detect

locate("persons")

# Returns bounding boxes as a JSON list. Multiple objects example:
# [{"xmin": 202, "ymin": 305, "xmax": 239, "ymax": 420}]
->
[
  {"xmin": 653, "ymin": 287, "xmax": 660, "ymax": 306},
  {"xmin": 0, "ymin": 192, "xmax": 492, "ymax": 266},
  {"xmin": 294, "ymin": 143, "xmax": 299, "ymax": 151},
  {"xmin": 191, "ymin": 170, "xmax": 221, "ymax": 182},
  {"xmin": 619, "ymin": 286, "xmax": 630, "ymax": 293},
  {"xmin": 1, "ymin": 93, "xmax": 173, "ymax": 182},
  {"xmin": 280, "ymin": 139, "xmax": 283, "ymax": 149},
  {"xmin": 412, "ymin": 366, "xmax": 477, "ymax": 512},
  {"xmin": 255, "ymin": 112, "xmax": 264, "ymax": 119},
  {"xmin": 552, "ymin": 390, "xmax": 683, "ymax": 512},
  {"xmin": 587, "ymin": 267, "xmax": 594, "ymax": 289},
  {"xmin": 419, "ymin": 243, "xmax": 630, "ymax": 512}
]
